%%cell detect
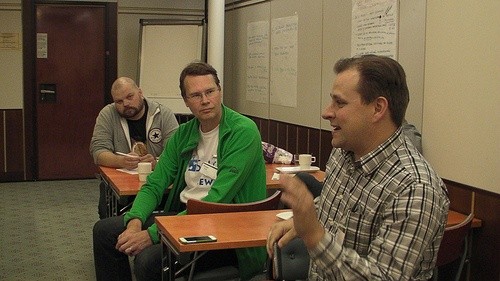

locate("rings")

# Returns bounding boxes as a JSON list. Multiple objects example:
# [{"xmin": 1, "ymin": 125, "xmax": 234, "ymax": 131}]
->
[{"xmin": 129, "ymin": 247, "xmax": 134, "ymax": 252}]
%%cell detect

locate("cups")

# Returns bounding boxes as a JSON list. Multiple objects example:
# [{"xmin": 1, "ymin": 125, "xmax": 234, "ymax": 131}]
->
[
  {"xmin": 138, "ymin": 162, "xmax": 151, "ymax": 182},
  {"xmin": 294, "ymin": 154, "xmax": 300, "ymax": 166},
  {"xmin": 298, "ymin": 154, "xmax": 315, "ymax": 169}
]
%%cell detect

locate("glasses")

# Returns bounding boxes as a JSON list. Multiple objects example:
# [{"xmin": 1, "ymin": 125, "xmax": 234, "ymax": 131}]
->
[{"xmin": 185, "ymin": 86, "xmax": 219, "ymax": 103}]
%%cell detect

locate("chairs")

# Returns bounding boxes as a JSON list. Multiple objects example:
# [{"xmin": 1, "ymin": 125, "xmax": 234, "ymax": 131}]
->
[
  {"xmin": 265, "ymin": 237, "xmax": 310, "ymax": 281},
  {"xmin": 431, "ymin": 213, "xmax": 475, "ymax": 281},
  {"xmin": 171, "ymin": 190, "xmax": 282, "ymax": 281}
]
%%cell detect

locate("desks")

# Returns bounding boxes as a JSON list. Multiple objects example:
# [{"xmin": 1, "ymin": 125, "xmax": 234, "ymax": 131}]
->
[
  {"xmin": 97, "ymin": 164, "xmax": 327, "ymax": 219},
  {"xmin": 154, "ymin": 208, "xmax": 482, "ymax": 281}
]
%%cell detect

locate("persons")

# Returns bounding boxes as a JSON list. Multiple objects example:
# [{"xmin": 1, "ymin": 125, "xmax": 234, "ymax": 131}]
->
[
  {"xmin": 91, "ymin": 60, "xmax": 268, "ymax": 281},
  {"xmin": 267, "ymin": 53, "xmax": 450, "ymax": 281},
  {"xmin": 276, "ymin": 102, "xmax": 422, "ymax": 210},
  {"xmin": 90, "ymin": 76, "xmax": 180, "ymax": 224}
]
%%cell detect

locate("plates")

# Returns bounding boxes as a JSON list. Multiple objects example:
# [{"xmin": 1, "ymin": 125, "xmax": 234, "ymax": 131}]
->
[
  {"xmin": 276, "ymin": 166, "xmax": 320, "ymax": 174},
  {"xmin": 277, "ymin": 211, "xmax": 293, "ymax": 220}
]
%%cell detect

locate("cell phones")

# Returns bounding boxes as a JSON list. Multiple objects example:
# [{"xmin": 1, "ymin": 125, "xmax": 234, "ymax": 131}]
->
[{"xmin": 178, "ymin": 236, "xmax": 218, "ymax": 244}]
